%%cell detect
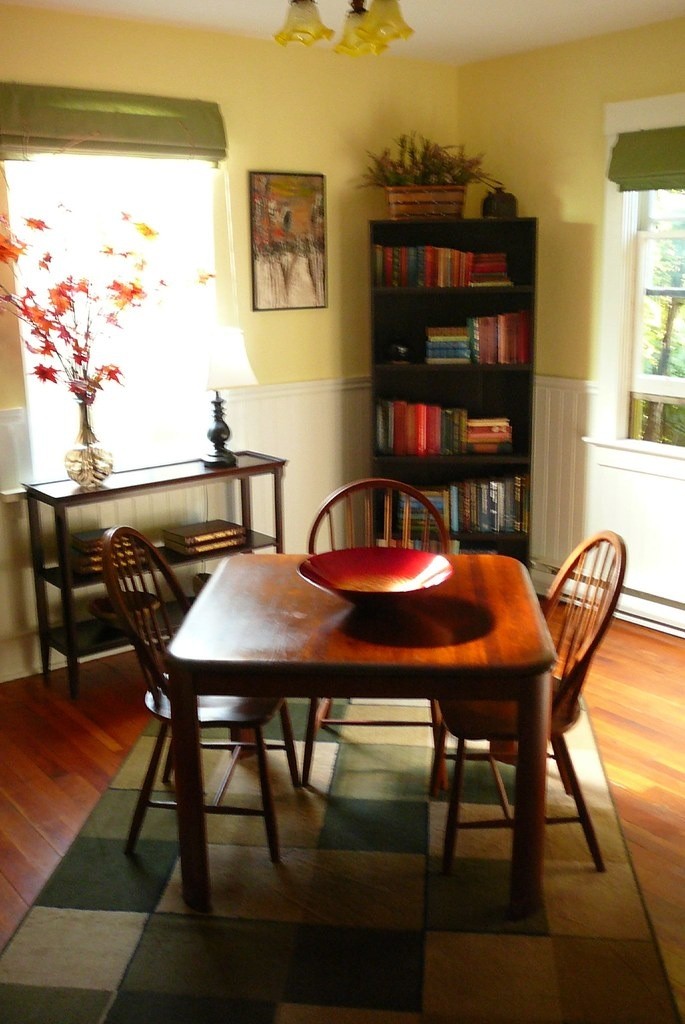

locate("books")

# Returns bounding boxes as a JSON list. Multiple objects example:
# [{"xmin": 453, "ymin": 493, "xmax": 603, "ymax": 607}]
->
[
  {"xmin": 67, "ymin": 517, "xmax": 250, "ymax": 577},
  {"xmin": 370, "ymin": 242, "xmax": 528, "ymax": 559}
]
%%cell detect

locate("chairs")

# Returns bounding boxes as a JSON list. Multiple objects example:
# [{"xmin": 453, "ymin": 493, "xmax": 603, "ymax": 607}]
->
[
  {"xmin": 303, "ymin": 479, "xmax": 451, "ymax": 792},
  {"xmin": 102, "ymin": 525, "xmax": 300, "ymax": 864},
  {"xmin": 429, "ymin": 531, "xmax": 627, "ymax": 877}
]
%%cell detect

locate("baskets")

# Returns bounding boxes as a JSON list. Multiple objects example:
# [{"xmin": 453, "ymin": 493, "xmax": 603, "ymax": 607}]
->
[{"xmin": 88, "ymin": 591, "xmax": 159, "ymax": 634}]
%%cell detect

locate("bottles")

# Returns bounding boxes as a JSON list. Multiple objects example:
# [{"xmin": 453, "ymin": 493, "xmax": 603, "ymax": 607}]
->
[{"xmin": 482, "ymin": 187, "xmax": 516, "ymax": 220}]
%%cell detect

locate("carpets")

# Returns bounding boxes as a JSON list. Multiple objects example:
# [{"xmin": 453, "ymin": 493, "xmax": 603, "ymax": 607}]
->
[{"xmin": 1, "ymin": 696, "xmax": 683, "ymax": 1024}]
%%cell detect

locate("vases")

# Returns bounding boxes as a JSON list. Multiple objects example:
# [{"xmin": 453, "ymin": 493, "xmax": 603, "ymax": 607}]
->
[
  {"xmin": 65, "ymin": 399, "xmax": 115, "ymax": 488},
  {"xmin": 386, "ymin": 185, "xmax": 465, "ymax": 219}
]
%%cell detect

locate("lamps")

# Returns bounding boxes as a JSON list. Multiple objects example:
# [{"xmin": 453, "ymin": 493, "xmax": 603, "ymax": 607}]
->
[
  {"xmin": 274, "ymin": 0, "xmax": 414, "ymax": 58},
  {"xmin": 200, "ymin": 324, "xmax": 259, "ymax": 469}
]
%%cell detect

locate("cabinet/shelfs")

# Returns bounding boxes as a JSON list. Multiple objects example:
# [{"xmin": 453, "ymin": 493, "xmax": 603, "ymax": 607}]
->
[
  {"xmin": 25, "ymin": 451, "xmax": 286, "ymax": 701},
  {"xmin": 368, "ymin": 217, "xmax": 537, "ymax": 573}
]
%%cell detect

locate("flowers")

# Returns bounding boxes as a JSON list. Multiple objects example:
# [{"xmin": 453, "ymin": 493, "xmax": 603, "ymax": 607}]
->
[{"xmin": 357, "ymin": 128, "xmax": 503, "ymax": 195}]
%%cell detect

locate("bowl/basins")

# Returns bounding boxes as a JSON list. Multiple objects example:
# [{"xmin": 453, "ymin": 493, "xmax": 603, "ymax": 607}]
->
[
  {"xmin": 298, "ymin": 547, "xmax": 453, "ymax": 610},
  {"xmin": 87, "ymin": 591, "xmax": 161, "ymax": 632}
]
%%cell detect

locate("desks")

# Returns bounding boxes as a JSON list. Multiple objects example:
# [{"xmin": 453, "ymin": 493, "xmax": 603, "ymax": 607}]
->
[{"xmin": 165, "ymin": 554, "xmax": 557, "ymax": 923}]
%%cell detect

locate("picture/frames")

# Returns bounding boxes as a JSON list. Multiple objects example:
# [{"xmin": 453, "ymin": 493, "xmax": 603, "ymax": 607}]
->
[{"xmin": 249, "ymin": 171, "xmax": 326, "ymax": 311}]
